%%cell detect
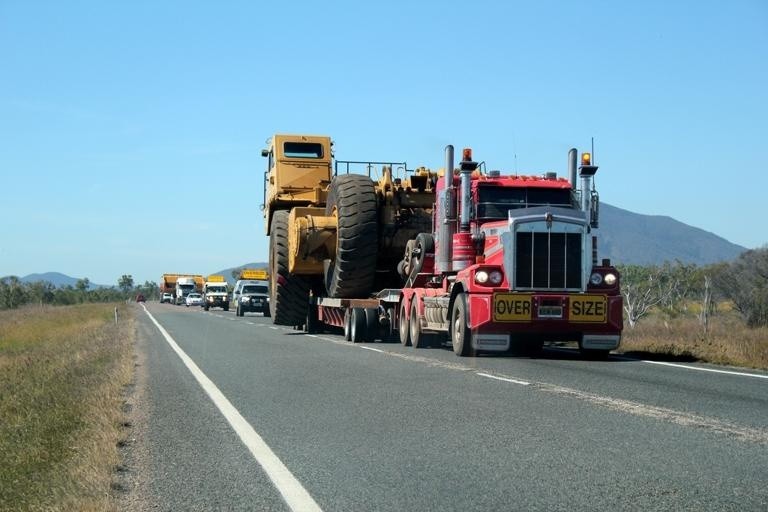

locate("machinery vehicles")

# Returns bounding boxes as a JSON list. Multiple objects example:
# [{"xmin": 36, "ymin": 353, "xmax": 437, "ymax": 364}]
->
[{"xmin": 260, "ymin": 135, "xmax": 479, "ymax": 326}]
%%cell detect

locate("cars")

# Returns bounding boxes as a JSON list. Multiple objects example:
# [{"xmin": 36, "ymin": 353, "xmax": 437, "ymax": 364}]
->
[
  {"xmin": 136, "ymin": 294, "xmax": 146, "ymax": 303},
  {"xmin": 160, "ymin": 274, "xmax": 205, "ymax": 307}
]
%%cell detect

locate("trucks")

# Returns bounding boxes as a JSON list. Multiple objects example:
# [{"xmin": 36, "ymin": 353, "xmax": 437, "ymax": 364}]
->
[
  {"xmin": 294, "ymin": 138, "xmax": 623, "ymax": 356},
  {"xmin": 203, "ymin": 276, "xmax": 229, "ymax": 311},
  {"xmin": 234, "ymin": 270, "xmax": 270, "ymax": 316}
]
